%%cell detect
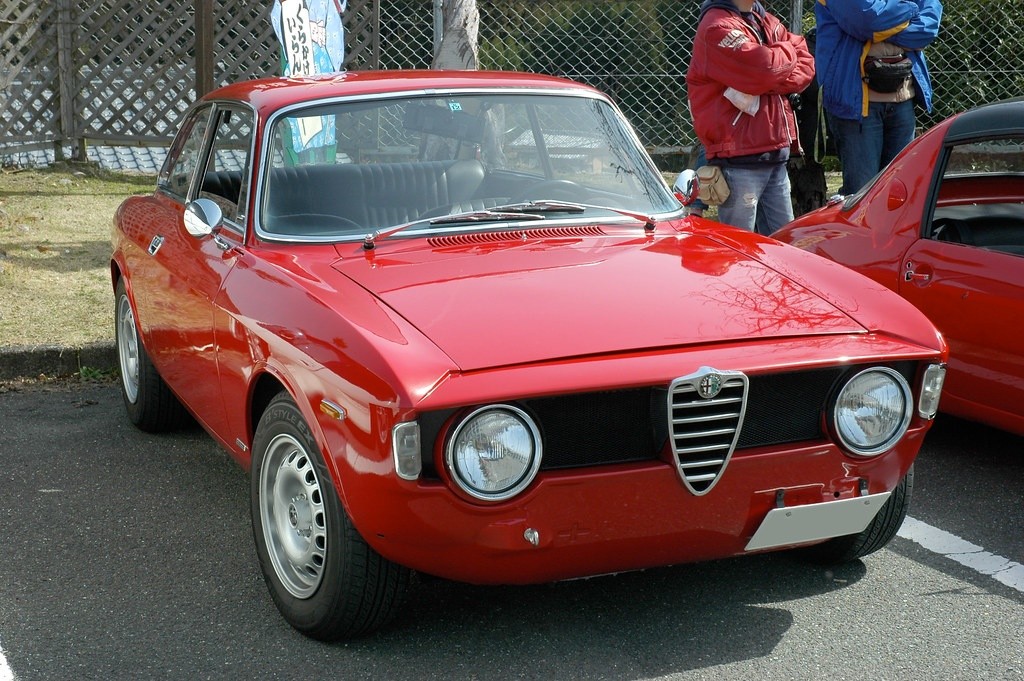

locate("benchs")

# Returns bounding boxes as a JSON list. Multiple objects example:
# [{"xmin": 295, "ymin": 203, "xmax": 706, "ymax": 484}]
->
[
  {"xmin": 936, "ymin": 215, "xmax": 1024, "ymax": 256},
  {"xmin": 197, "ymin": 159, "xmax": 490, "ymax": 231}
]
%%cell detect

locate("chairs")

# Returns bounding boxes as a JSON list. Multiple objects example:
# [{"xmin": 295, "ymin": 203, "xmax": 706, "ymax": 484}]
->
[
  {"xmin": 419, "ymin": 197, "xmax": 512, "ymax": 220},
  {"xmin": 267, "ymin": 214, "xmax": 362, "ymax": 230}
]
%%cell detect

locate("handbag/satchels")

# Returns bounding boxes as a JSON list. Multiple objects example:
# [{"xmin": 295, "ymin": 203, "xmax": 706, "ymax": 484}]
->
[
  {"xmin": 861, "ymin": 54, "xmax": 913, "ymax": 94},
  {"xmin": 696, "ymin": 166, "xmax": 730, "ymax": 206}
]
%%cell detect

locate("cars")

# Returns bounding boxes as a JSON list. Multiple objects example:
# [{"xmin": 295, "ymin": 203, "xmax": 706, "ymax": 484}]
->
[
  {"xmin": 108, "ymin": 71, "xmax": 949, "ymax": 644},
  {"xmin": 759, "ymin": 95, "xmax": 1024, "ymax": 439}
]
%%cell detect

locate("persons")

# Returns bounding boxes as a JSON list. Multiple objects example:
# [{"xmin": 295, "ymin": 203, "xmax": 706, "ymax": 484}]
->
[
  {"xmin": 688, "ymin": 0, "xmax": 816, "ymax": 239},
  {"xmin": 690, "ymin": 142, "xmax": 710, "ymax": 219},
  {"xmin": 814, "ymin": 0, "xmax": 942, "ymax": 197}
]
